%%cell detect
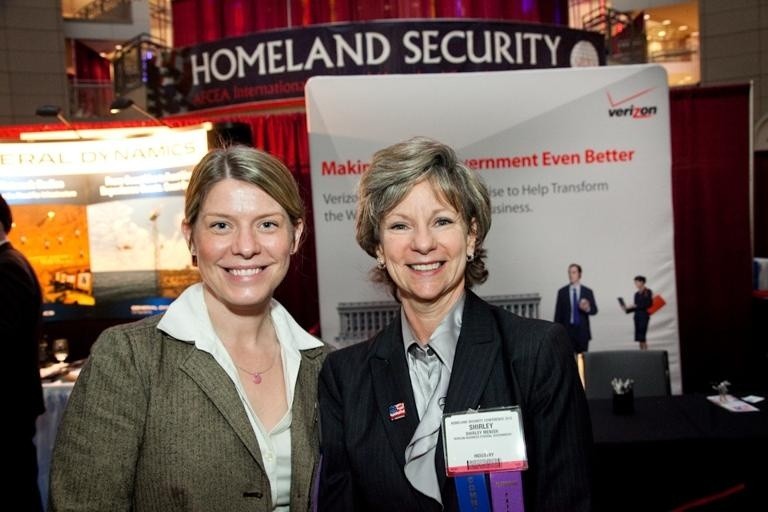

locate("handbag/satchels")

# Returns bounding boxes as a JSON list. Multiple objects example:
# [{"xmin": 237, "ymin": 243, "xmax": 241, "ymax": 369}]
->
[{"xmin": 646, "ymin": 294, "xmax": 666, "ymax": 315}]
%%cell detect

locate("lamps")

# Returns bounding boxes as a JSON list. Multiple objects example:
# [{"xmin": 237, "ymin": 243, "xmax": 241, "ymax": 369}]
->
[
  {"xmin": 36, "ymin": 106, "xmax": 81, "ymax": 138},
  {"xmin": 109, "ymin": 98, "xmax": 170, "ymax": 129}
]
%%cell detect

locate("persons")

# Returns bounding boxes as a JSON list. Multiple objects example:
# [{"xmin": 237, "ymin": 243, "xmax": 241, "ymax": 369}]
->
[
  {"xmin": 0, "ymin": 195, "xmax": 46, "ymax": 512},
  {"xmin": 48, "ymin": 146, "xmax": 338, "ymax": 511},
  {"xmin": 620, "ymin": 276, "xmax": 653, "ymax": 351},
  {"xmin": 317, "ymin": 136, "xmax": 596, "ymax": 512},
  {"xmin": 555, "ymin": 264, "xmax": 597, "ymax": 352}
]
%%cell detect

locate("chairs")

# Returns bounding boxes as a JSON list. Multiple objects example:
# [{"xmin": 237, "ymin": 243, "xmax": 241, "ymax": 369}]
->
[{"xmin": 581, "ymin": 349, "xmax": 670, "ymax": 395}]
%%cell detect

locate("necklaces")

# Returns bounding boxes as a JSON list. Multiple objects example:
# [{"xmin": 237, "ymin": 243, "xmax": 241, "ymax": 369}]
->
[{"xmin": 235, "ymin": 351, "xmax": 277, "ymax": 384}]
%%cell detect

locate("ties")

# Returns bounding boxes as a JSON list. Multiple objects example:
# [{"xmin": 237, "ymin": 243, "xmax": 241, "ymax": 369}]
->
[{"xmin": 573, "ymin": 288, "xmax": 579, "ymax": 327}]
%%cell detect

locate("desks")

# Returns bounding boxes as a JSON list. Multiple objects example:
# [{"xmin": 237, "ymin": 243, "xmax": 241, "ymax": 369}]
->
[{"xmin": 588, "ymin": 393, "xmax": 767, "ymax": 512}]
